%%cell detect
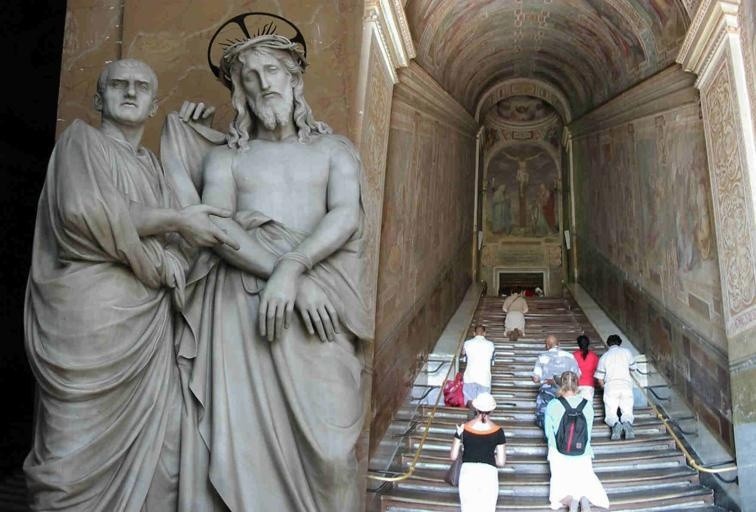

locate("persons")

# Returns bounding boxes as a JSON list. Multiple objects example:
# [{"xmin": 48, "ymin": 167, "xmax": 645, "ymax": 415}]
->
[
  {"xmin": 593, "ymin": 334, "xmax": 638, "ymax": 440},
  {"xmin": 572, "ymin": 334, "xmax": 600, "ymax": 401},
  {"xmin": 24, "ymin": 57, "xmax": 241, "ymax": 512},
  {"xmin": 492, "ymin": 157, "xmax": 556, "ymax": 237},
  {"xmin": 531, "ymin": 333, "xmax": 583, "ymax": 428},
  {"xmin": 202, "ymin": 33, "xmax": 376, "ymax": 512},
  {"xmin": 543, "ymin": 371, "xmax": 609, "ymax": 512},
  {"xmin": 449, "ymin": 392, "xmax": 506, "ymax": 512},
  {"xmin": 502, "ymin": 288, "xmax": 528, "ymax": 340},
  {"xmin": 462, "ymin": 325, "xmax": 496, "ymax": 416}
]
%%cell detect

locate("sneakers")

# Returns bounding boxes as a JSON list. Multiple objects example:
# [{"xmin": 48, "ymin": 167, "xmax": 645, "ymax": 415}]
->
[
  {"xmin": 569, "ymin": 497, "xmax": 591, "ymax": 512},
  {"xmin": 610, "ymin": 422, "xmax": 634, "ymax": 441},
  {"xmin": 509, "ymin": 329, "xmax": 519, "ymax": 341}
]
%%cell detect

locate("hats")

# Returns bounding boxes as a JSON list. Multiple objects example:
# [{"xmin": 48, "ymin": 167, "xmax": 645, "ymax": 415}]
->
[{"xmin": 472, "ymin": 392, "xmax": 497, "ymax": 412}]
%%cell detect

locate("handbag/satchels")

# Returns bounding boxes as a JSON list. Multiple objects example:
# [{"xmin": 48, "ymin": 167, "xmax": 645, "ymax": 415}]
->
[
  {"xmin": 445, "ymin": 446, "xmax": 463, "ymax": 487},
  {"xmin": 443, "ymin": 373, "xmax": 464, "ymax": 409}
]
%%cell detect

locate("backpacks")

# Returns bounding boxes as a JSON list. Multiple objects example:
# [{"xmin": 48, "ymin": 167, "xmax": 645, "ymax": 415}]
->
[{"xmin": 555, "ymin": 396, "xmax": 588, "ymax": 456}]
